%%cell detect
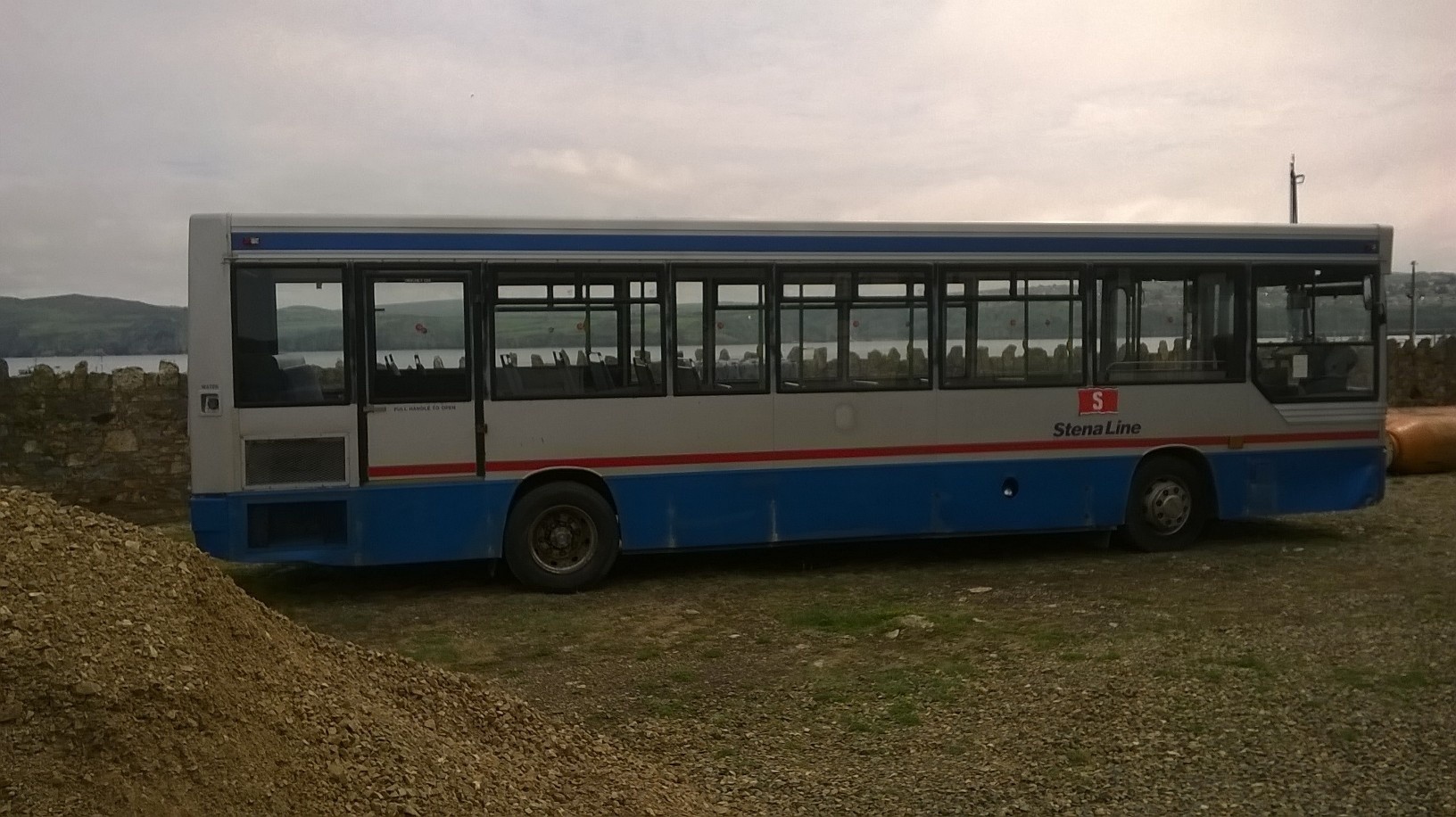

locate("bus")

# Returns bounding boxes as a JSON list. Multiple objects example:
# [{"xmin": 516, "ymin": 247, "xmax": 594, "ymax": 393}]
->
[{"xmin": 185, "ymin": 210, "xmax": 1393, "ymax": 595}]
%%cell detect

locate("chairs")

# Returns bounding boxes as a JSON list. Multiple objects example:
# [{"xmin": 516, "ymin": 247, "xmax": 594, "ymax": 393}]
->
[{"xmin": 282, "ymin": 337, "xmax": 1218, "ymax": 403}]
